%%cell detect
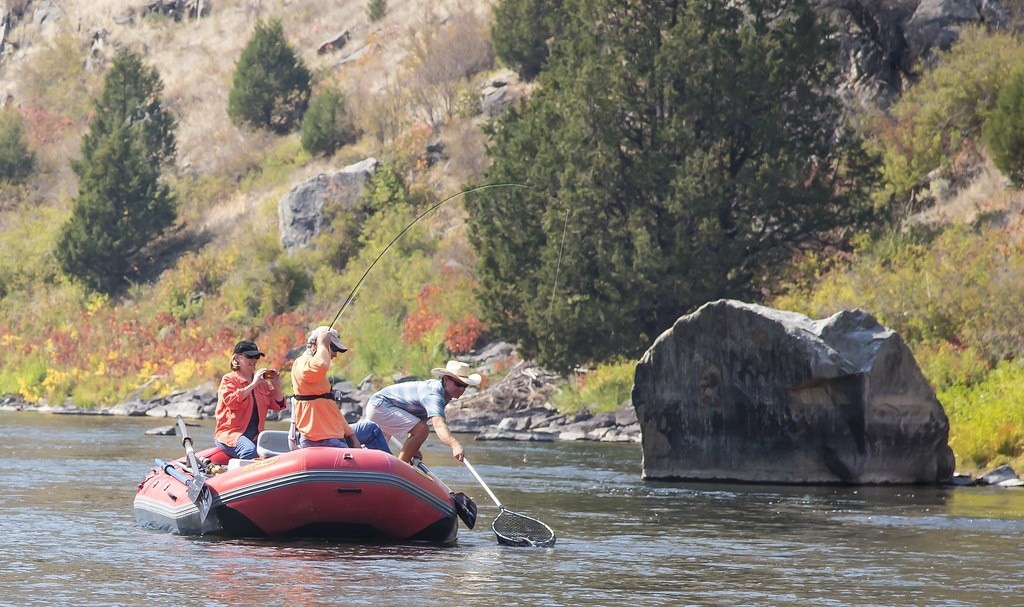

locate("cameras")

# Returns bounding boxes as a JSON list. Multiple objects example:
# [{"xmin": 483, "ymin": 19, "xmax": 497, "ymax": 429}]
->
[{"xmin": 263, "ymin": 370, "xmax": 274, "ymax": 379}]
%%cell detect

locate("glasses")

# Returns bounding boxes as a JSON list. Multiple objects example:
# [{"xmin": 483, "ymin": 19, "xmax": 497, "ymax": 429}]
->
[
  {"xmin": 330, "ymin": 344, "xmax": 338, "ymax": 352},
  {"xmin": 449, "ymin": 378, "xmax": 468, "ymax": 388},
  {"xmin": 240, "ymin": 354, "xmax": 260, "ymax": 359}
]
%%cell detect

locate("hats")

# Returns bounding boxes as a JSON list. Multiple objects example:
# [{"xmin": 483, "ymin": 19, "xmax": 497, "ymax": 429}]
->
[
  {"xmin": 311, "ymin": 326, "xmax": 348, "ymax": 353},
  {"xmin": 431, "ymin": 360, "xmax": 482, "ymax": 386},
  {"xmin": 234, "ymin": 341, "xmax": 265, "ymax": 357}
]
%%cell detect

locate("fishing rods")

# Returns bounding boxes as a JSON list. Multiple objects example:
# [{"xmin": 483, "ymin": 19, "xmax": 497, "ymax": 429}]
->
[{"xmin": 326, "ymin": 184, "xmax": 571, "ymax": 333}]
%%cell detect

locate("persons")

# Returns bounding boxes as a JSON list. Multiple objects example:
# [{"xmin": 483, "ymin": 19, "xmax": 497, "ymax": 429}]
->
[
  {"xmin": 365, "ymin": 361, "xmax": 482, "ymax": 463},
  {"xmin": 213, "ymin": 340, "xmax": 287, "ymax": 459},
  {"xmin": 291, "ymin": 326, "xmax": 392, "ymax": 454}
]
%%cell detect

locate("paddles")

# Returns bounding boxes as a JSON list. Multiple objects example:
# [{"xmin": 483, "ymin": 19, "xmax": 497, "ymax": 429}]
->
[{"xmin": 153, "ymin": 414, "xmax": 214, "ymax": 524}]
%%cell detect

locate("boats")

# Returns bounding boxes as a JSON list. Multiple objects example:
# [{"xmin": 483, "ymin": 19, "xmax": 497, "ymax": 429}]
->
[{"xmin": 132, "ymin": 430, "xmax": 460, "ymax": 547}]
